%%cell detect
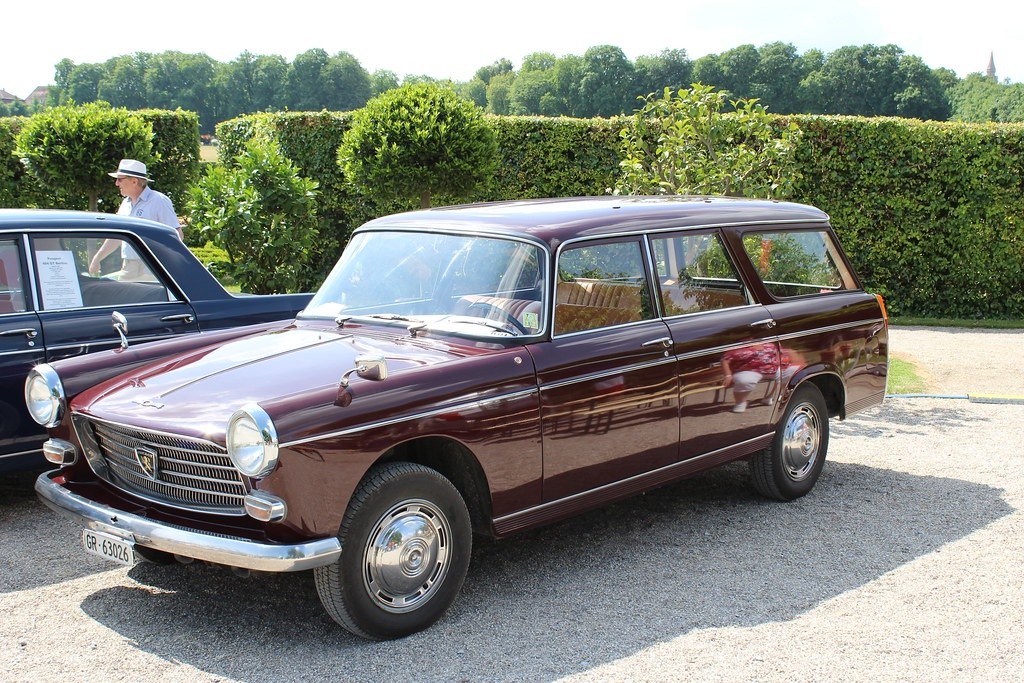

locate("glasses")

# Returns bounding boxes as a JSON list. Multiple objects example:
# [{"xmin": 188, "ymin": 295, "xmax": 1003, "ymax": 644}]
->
[{"xmin": 113, "ymin": 177, "xmax": 128, "ymax": 182}]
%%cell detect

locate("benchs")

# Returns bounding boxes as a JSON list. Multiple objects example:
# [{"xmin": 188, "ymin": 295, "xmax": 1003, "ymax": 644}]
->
[{"xmin": 556, "ymin": 281, "xmax": 747, "ymax": 316}]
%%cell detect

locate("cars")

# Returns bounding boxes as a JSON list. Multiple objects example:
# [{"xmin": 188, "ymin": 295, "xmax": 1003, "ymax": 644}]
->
[
  {"xmin": 21, "ymin": 191, "xmax": 890, "ymax": 643},
  {"xmin": 0, "ymin": 206, "xmax": 318, "ymax": 477}
]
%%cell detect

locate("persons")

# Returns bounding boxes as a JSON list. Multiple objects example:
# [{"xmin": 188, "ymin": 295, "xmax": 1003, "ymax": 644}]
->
[
  {"xmin": 88, "ymin": 159, "xmax": 183, "ymax": 282},
  {"xmin": 722, "ymin": 337, "xmax": 806, "ymax": 412}
]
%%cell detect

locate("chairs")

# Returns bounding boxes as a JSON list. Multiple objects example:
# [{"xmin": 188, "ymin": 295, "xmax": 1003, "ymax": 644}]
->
[
  {"xmin": 453, "ymin": 295, "xmax": 541, "ymax": 331},
  {"xmin": 80, "ymin": 280, "xmax": 168, "ymax": 307},
  {"xmin": 555, "ymin": 303, "xmax": 641, "ymax": 336}
]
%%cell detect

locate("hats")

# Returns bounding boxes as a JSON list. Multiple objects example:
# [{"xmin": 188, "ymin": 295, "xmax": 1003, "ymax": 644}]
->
[{"xmin": 107, "ymin": 158, "xmax": 155, "ymax": 185}]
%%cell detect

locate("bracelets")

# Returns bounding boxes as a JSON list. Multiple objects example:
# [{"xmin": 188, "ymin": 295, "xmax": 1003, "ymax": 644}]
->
[{"xmin": 725, "ymin": 374, "xmax": 731, "ymax": 377}]
